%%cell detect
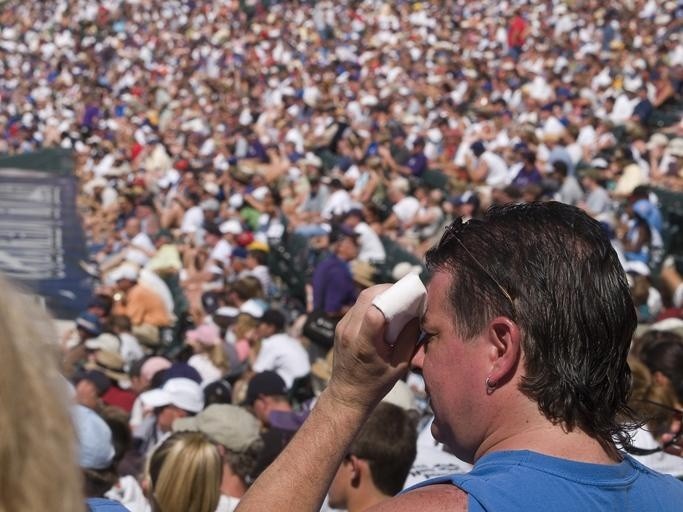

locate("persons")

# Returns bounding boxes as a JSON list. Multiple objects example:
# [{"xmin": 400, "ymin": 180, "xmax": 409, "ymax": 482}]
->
[{"xmin": 1, "ymin": 0, "xmax": 681, "ymax": 511}]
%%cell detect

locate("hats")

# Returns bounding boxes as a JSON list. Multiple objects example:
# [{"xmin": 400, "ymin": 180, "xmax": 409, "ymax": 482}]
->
[{"xmin": 71, "ymin": 266, "xmax": 421, "ymax": 471}]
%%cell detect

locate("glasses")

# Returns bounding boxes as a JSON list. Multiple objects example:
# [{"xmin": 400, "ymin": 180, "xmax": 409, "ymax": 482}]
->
[{"xmin": 436, "ymin": 216, "xmax": 518, "ymax": 322}]
